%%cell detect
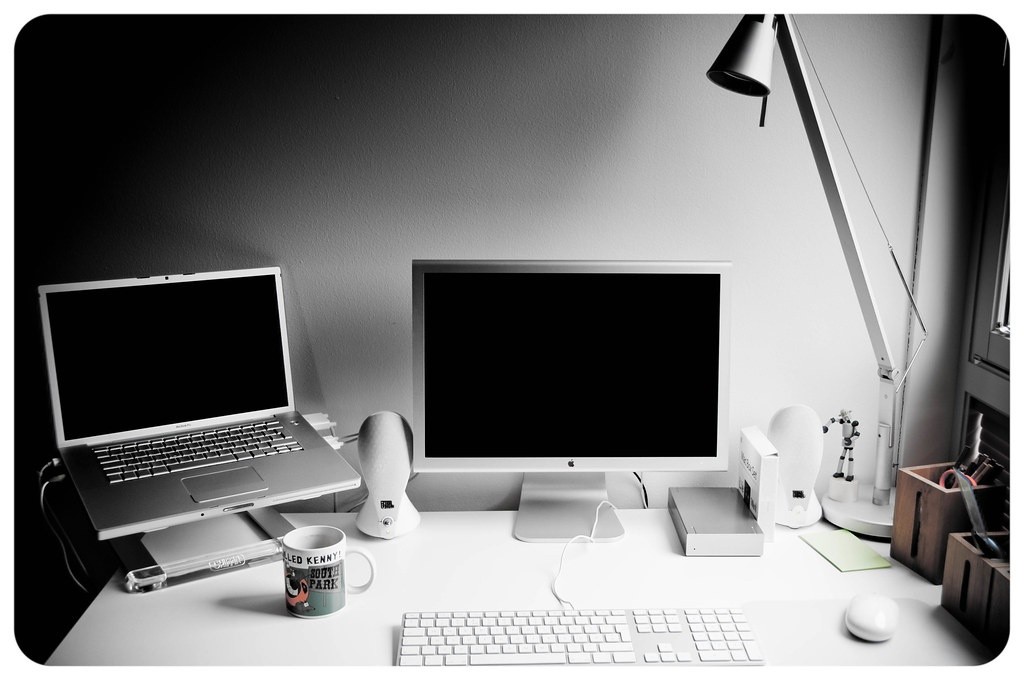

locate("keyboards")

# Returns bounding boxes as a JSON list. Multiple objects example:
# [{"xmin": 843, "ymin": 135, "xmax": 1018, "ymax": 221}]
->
[{"xmin": 396, "ymin": 609, "xmax": 765, "ymax": 670}]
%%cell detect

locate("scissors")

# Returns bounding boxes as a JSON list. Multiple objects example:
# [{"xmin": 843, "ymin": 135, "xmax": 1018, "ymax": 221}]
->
[{"xmin": 940, "ymin": 470, "xmax": 977, "ymax": 489}]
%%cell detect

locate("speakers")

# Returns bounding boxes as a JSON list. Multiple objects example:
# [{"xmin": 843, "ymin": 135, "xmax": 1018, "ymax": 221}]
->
[
  {"xmin": 356, "ymin": 410, "xmax": 420, "ymax": 542},
  {"xmin": 770, "ymin": 406, "xmax": 822, "ymax": 530}
]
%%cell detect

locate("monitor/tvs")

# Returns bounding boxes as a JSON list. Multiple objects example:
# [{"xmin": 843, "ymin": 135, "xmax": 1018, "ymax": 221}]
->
[{"xmin": 411, "ymin": 259, "xmax": 731, "ymax": 541}]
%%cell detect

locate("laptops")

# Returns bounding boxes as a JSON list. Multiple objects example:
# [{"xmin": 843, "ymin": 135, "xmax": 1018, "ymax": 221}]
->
[{"xmin": 39, "ymin": 266, "xmax": 361, "ymax": 542}]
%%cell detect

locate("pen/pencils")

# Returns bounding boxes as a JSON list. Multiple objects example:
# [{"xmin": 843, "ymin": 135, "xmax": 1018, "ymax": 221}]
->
[{"xmin": 965, "ymin": 453, "xmax": 1004, "ymax": 485}]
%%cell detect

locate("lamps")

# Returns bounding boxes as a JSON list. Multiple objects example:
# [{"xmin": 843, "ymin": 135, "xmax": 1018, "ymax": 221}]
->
[{"xmin": 706, "ymin": 14, "xmax": 928, "ymax": 543}]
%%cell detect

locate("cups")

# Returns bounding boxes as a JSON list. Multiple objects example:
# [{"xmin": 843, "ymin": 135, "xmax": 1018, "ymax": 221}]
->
[{"xmin": 283, "ymin": 525, "xmax": 374, "ymax": 618}]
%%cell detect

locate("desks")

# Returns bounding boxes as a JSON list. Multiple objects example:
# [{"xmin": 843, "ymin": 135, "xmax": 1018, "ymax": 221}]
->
[{"xmin": 42, "ymin": 509, "xmax": 996, "ymax": 667}]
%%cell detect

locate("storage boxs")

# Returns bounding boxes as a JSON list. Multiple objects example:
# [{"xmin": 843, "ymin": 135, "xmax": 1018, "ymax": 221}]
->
[{"xmin": 737, "ymin": 427, "xmax": 779, "ymax": 542}]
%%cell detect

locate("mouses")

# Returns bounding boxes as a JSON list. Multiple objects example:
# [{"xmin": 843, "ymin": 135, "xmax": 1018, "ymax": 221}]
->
[{"xmin": 845, "ymin": 595, "xmax": 900, "ymax": 642}]
